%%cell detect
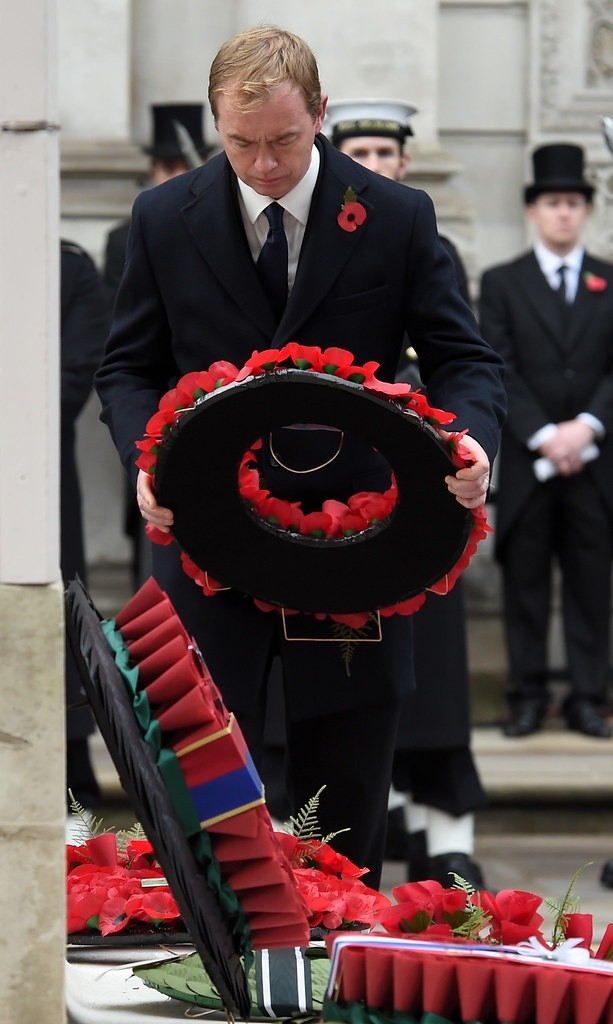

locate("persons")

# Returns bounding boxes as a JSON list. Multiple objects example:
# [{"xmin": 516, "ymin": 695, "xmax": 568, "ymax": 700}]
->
[
  {"xmin": 93, "ymin": 25, "xmax": 511, "ymax": 954},
  {"xmin": 59, "ymin": 103, "xmax": 470, "ymax": 806},
  {"xmin": 477, "ymin": 143, "xmax": 613, "ymax": 739}
]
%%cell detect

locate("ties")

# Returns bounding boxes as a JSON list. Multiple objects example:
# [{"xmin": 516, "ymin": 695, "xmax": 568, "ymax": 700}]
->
[
  {"xmin": 256, "ymin": 204, "xmax": 289, "ymax": 303},
  {"xmin": 556, "ymin": 266, "xmax": 570, "ymax": 296}
]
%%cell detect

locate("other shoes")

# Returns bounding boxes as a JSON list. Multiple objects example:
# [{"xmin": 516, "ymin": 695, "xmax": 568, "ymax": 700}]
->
[
  {"xmin": 568, "ymin": 701, "xmax": 604, "ymax": 734},
  {"xmin": 510, "ymin": 696, "xmax": 545, "ymax": 736},
  {"xmin": 427, "ymin": 851, "xmax": 484, "ymax": 892}
]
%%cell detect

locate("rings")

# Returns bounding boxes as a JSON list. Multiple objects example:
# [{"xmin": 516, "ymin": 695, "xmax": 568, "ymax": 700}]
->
[{"xmin": 484, "ymin": 478, "xmax": 489, "ymax": 484}]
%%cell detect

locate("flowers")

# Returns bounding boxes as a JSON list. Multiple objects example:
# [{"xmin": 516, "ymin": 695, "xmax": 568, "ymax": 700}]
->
[
  {"xmin": 63, "ymin": 574, "xmax": 613, "ymax": 1024},
  {"xmin": 131, "ymin": 341, "xmax": 494, "ymax": 678},
  {"xmin": 336, "ymin": 185, "xmax": 368, "ymax": 233},
  {"xmin": 582, "ymin": 271, "xmax": 607, "ymax": 292}
]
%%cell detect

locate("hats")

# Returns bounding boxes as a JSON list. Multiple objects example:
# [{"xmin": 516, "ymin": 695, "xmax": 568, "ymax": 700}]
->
[
  {"xmin": 323, "ymin": 99, "xmax": 417, "ymax": 142},
  {"xmin": 523, "ymin": 143, "xmax": 595, "ymax": 204},
  {"xmin": 140, "ymin": 104, "xmax": 216, "ymax": 157}
]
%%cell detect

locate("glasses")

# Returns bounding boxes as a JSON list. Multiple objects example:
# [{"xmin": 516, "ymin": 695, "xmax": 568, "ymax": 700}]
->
[{"xmin": 161, "ymin": 160, "xmax": 190, "ymax": 172}]
